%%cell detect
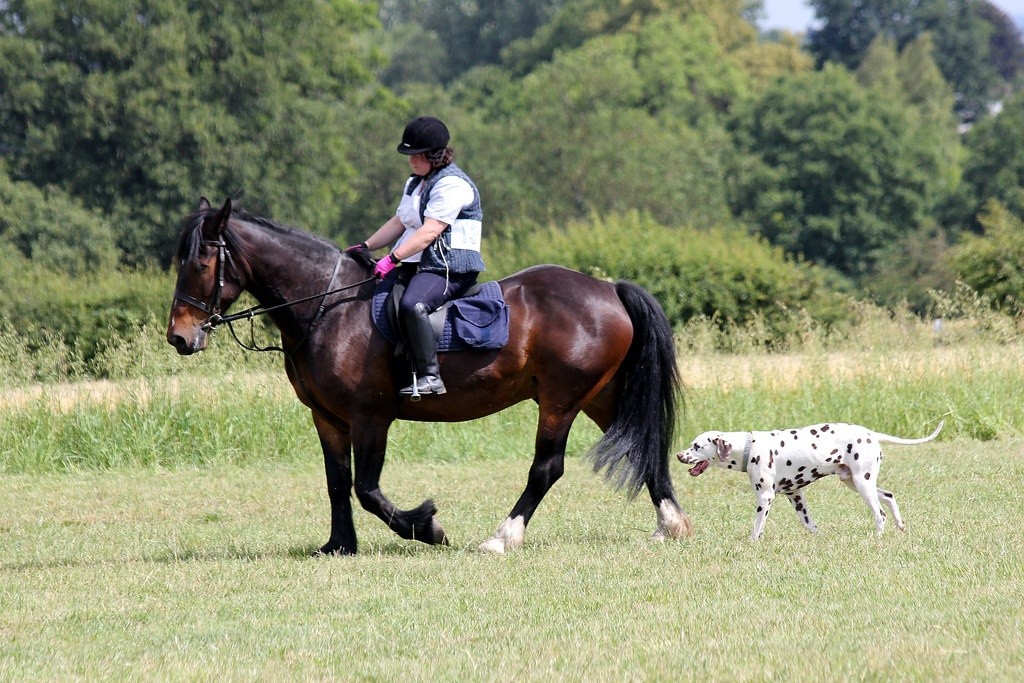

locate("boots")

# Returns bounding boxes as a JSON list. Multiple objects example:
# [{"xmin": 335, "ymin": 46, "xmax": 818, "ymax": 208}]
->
[{"xmin": 399, "ymin": 302, "xmax": 447, "ymax": 395}]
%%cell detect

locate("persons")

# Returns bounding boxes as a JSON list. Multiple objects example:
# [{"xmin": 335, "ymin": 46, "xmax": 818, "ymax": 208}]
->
[{"xmin": 345, "ymin": 116, "xmax": 484, "ymax": 395}]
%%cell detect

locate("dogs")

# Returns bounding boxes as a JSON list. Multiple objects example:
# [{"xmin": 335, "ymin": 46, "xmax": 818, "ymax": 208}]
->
[{"xmin": 677, "ymin": 420, "xmax": 944, "ymax": 540}]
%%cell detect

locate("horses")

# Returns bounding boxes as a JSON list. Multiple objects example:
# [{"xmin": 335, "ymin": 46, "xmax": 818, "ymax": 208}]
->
[{"xmin": 165, "ymin": 197, "xmax": 698, "ymax": 558}]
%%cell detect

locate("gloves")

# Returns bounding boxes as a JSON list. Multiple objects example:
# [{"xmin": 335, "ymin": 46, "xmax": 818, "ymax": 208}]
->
[
  {"xmin": 344, "ymin": 243, "xmax": 368, "ymax": 253},
  {"xmin": 375, "ymin": 252, "xmax": 400, "ymax": 280}
]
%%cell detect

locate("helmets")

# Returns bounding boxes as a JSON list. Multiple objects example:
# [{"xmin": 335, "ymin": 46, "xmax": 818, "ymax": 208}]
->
[{"xmin": 398, "ymin": 117, "xmax": 449, "ymax": 155}]
